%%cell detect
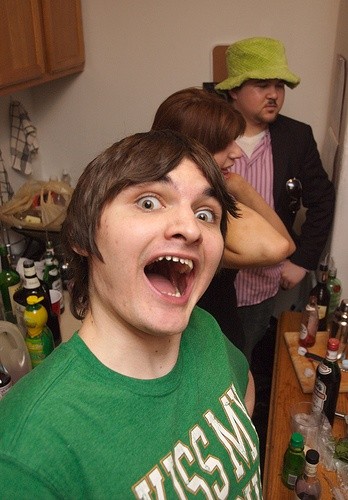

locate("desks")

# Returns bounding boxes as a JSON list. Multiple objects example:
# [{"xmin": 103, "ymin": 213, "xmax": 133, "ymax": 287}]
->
[{"xmin": 262, "ymin": 311, "xmax": 348, "ymax": 500}]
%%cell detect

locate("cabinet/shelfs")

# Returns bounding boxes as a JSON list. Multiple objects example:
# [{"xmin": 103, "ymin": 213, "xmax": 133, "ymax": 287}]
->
[{"xmin": 0, "ymin": 0, "xmax": 85, "ymax": 97}]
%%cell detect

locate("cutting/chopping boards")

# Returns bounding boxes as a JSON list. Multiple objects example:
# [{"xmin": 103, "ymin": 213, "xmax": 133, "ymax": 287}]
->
[{"xmin": 283, "ymin": 330, "xmax": 348, "ymax": 394}]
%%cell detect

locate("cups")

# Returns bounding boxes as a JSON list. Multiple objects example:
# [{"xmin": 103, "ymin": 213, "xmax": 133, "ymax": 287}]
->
[
  {"xmin": 291, "ymin": 402, "xmax": 325, "ymax": 455},
  {"xmin": 50, "ymin": 290, "xmax": 62, "ymax": 327}
]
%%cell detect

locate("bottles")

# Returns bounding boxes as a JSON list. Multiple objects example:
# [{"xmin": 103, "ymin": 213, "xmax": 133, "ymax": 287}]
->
[
  {"xmin": 310, "ymin": 338, "xmax": 341, "ymax": 429},
  {"xmin": 329, "ymin": 299, "xmax": 348, "ymax": 360},
  {"xmin": 60, "ymin": 171, "xmax": 71, "ymax": 203},
  {"xmin": 307, "ymin": 262, "xmax": 330, "ymax": 331},
  {"xmin": 299, "ymin": 295, "xmax": 319, "ymax": 347},
  {"xmin": 0, "ymin": 240, "xmax": 65, "ymax": 399},
  {"xmin": 281, "ymin": 432, "xmax": 306, "ymax": 489},
  {"xmin": 326, "ymin": 267, "xmax": 342, "ymax": 322},
  {"xmin": 290, "ymin": 449, "xmax": 321, "ymax": 500}
]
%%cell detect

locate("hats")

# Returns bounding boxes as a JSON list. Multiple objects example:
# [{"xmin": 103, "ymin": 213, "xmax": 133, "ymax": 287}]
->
[{"xmin": 214, "ymin": 36, "xmax": 301, "ymax": 90}]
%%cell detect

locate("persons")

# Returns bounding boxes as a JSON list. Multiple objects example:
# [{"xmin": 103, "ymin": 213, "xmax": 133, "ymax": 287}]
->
[
  {"xmin": 0, "ymin": 130, "xmax": 263, "ymax": 500},
  {"xmin": 151, "ymin": 37, "xmax": 336, "ymax": 367}
]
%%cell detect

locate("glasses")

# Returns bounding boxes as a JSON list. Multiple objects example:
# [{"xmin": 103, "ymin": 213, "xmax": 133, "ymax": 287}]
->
[{"xmin": 286, "ymin": 177, "xmax": 301, "ymax": 215}]
